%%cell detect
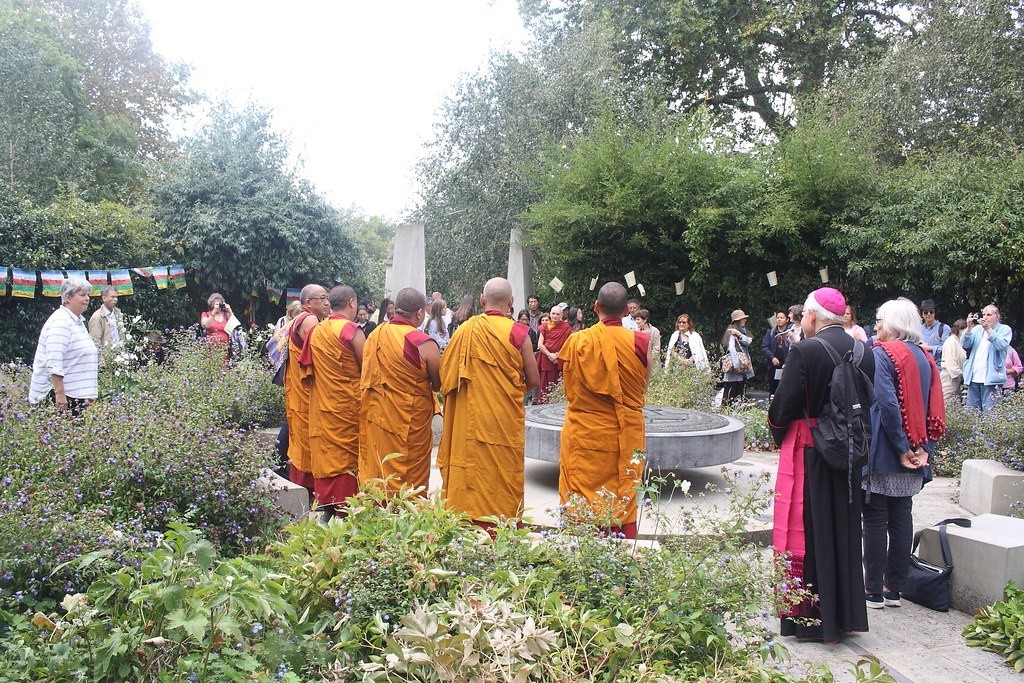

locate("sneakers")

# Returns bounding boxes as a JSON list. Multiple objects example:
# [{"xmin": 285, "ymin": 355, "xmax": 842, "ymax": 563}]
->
[
  {"xmin": 881, "ymin": 589, "xmax": 901, "ymax": 607},
  {"xmin": 866, "ymin": 592, "xmax": 885, "ymax": 609}
]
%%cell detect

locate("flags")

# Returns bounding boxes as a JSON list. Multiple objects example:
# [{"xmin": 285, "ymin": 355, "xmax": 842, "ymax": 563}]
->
[{"xmin": 0, "ymin": 264, "xmax": 187, "ymax": 300}]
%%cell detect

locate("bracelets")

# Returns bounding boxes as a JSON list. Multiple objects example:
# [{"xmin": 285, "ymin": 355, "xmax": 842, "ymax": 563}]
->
[{"xmin": 211, "ymin": 312, "xmax": 216, "ymax": 315}]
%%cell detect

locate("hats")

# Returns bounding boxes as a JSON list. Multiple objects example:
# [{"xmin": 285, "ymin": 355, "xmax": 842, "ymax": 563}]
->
[
  {"xmin": 919, "ymin": 300, "xmax": 937, "ymax": 310},
  {"xmin": 807, "ymin": 287, "xmax": 847, "ymax": 322}
]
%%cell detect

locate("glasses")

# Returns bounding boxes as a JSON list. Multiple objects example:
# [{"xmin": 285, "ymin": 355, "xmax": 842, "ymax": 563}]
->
[
  {"xmin": 307, "ymin": 296, "xmax": 329, "ymax": 302},
  {"xmin": 676, "ymin": 321, "xmax": 688, "ymax": 324},
  {"xmin": 922, "ymin": 309, "xmax": 935, "ymax": 315},
  {"xmin": 875, "ymin": 318, "xmax": 883, "ymax": 325}
]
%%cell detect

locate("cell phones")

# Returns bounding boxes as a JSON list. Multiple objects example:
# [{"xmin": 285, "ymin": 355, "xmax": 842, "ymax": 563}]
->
[
  {"xmin": 219, "ymin": 304, "xmax": 225, "ymax": 308},
  {"xmin": 972, "ymin": 313, "xmax": 983, "ymax": 319}
]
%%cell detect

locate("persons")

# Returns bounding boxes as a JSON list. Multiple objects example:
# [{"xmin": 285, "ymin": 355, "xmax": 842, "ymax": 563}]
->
[
  {"xmin": 132, "ymin": 330, "xmax": 176, "ymax": 369},
  {"xmin": 201, "ymin": 292, "xmax": 235, "ymax": 371},
  {"xmin": 762, "ymin": 287, "xmax": 879, "ymax": 640},
  {"xmin": 25, "ymin": 274, "xmax": 100, "ymax": 430},
  {"xmin": 88, "ymin": 285, "xmax": 128, "ymax": 366},
  {"xmin": 861, "ymin": 298, "xmax": 948, "ymax": 610},
  {"xmin": 256, "ymin": 279, "xmax": 1022, "ymax": 570}
]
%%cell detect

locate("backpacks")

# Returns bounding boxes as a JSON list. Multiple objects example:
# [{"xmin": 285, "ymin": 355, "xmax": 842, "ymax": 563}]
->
[{"xmin": 807, "ymin": 336, "xmax": 876, "ymax": 504}]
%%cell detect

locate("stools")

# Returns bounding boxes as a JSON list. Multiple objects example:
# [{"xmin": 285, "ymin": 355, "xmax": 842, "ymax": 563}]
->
[
  {"xmin": 256, "ymin": 467, "xmax": 309, "ymax": 525},
  {"xmin": 958, "ymin": 458, "xmax": 1024, "ymax": 516},
  {"xmin": 920, "ymin": 513, "xmax": 1024, "ymax": 622}
]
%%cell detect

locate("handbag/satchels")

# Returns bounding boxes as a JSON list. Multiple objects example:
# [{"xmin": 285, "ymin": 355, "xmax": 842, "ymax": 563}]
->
[
  {"xmin": 721, "ymin": 351, "xmax": 753, "ymax": 375},
  {"xmin": 899, "ymin": 553, "xmax": 955, "ymax": 613}
]
